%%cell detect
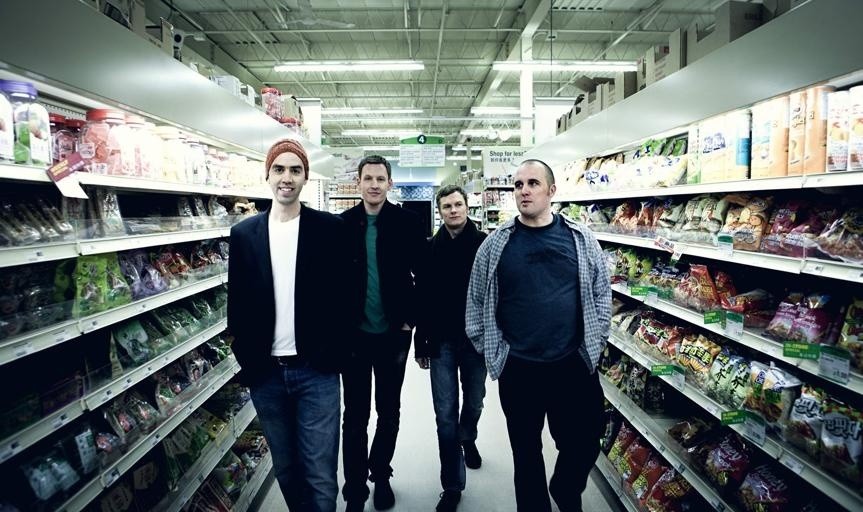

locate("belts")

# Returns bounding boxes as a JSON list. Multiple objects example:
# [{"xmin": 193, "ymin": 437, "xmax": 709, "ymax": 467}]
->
[{"xmin": 267, "ymin": 358, "xmax": 296, "ymax": 366}]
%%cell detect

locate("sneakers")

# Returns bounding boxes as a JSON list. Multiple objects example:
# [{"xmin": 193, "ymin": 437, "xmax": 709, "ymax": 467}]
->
[
  {"xmin": 374, "ymin": 479, "xmax": 395, "ymax": 510},
  {"xmin": 436, "ymin": 489, "xmax": 461, "ymax": 512},
  {"xmin": 464, "ymin": 441, "xmax": 481, "ymax": 468},
  {"xmin": 346, "ymin": 499, "xmax": 364, "ymax": 512}
]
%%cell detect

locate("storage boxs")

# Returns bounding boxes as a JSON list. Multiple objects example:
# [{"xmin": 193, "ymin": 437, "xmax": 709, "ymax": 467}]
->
[{"xmin": 556, "ymin": 0, "xmax": 803, "ymax": 136}]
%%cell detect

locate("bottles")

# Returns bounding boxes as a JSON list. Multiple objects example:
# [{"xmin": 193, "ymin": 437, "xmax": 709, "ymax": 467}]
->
[{"xmin": 0, "ymin": 82, "xmax": 232, "ymax": 188}]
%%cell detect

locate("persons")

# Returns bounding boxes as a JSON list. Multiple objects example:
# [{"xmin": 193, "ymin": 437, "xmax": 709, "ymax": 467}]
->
[
  {"xmin": 339, "ymin": 155, "xmax": 433, "ymax": 512},
  {"xmin": 414, "ymin": 185, "xmax": 488, "ymax": 512},
  {"xmin": 466, "ymin": 159, "xmax": 612, "ymax": 512},
  {"xmin": 227, "ymin": 139, "xmax": 360, "ymax": 512}
]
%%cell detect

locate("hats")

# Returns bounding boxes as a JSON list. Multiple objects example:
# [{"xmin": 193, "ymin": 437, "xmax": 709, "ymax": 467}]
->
[{"xmin": 266, "ymin": 139, "xmax": 309, "ymax": 180}]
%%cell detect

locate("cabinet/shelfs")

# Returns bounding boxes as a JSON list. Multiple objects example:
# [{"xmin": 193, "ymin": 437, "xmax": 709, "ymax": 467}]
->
[
  {"xmin": 470, "ymin": 167, "xmax": 863, "ymax": 512},
  {"xmin": 0, "ymin": 61, "xmax": 277, "ymax": 512}
]
